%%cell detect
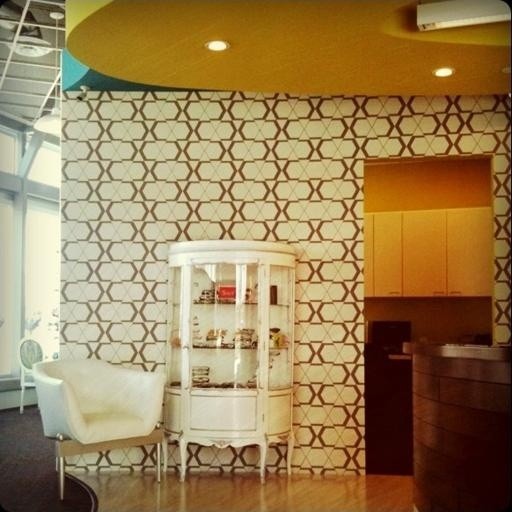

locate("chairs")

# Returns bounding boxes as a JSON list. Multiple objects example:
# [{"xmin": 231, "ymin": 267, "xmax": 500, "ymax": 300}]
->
[{"xmin": 19, "ymin": 338, "xmax": 44, "ymax": 416}]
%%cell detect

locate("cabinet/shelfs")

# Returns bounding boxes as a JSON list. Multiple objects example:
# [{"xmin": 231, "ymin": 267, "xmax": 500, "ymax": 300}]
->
[{"xmin": 163, "ymin": 238, "xmax": 295, "ymax": 483}]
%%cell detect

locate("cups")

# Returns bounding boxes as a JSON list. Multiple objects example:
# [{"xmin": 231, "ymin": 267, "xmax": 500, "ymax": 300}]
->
[{"xmin": 403, "ymin": 342, "xmax": 411, "ymax": 354}]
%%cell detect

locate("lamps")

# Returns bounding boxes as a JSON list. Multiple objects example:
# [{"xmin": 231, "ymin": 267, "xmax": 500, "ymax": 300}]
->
[
  {"xmin": 0, "ymin": 0, "xmax": 40, "ymax": 33},
  {"xmin": 3, "ymin": 32, "xmax": 60, "ymax": 64},
  {"xmin": 2, "ymin": 28, "xmax": 53, "ymax": 59}
]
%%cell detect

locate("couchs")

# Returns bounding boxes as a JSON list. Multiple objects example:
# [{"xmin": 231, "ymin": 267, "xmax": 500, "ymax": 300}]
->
[{"xmin": 32, "ymin": 357, "xmax": 168, "ymax": 500}]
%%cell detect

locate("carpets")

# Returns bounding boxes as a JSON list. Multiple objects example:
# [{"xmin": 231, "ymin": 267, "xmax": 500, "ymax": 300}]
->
[{"xmin": 1, "ymin": 404, "xmax": 100, "ymax": 511}]
